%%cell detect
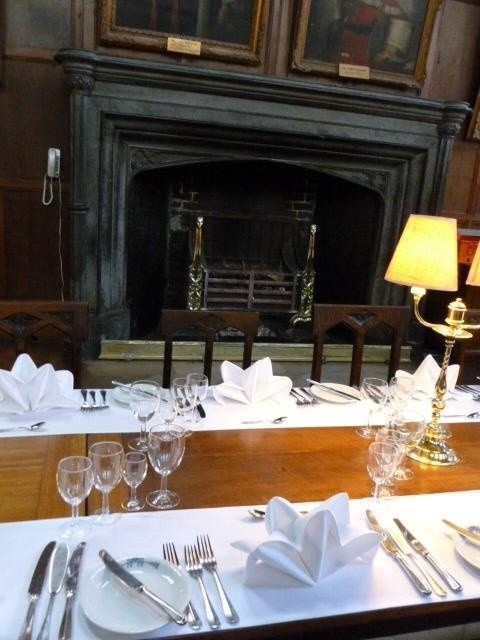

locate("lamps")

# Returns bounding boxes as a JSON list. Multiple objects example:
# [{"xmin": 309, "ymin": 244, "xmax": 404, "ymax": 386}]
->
[{"xmin": 383, "ymin": 212, "xmax": 480, "ymax": 466}]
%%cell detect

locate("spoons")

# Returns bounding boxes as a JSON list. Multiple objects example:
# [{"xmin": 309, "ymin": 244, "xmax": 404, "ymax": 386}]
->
[
  {"xmin": 437, "ymin": 410, "xmax": 480, "ymax": 422},
  {"xmin": 0, "ymin": 420, "xmax": 48, "ymax": 433},
  {"xmin": 239, "ymin": 416, "xmax": 291, "ymax": 427},
  {"xmin": 247, "ymin": 508, "xmax": 312, "ymax": 521}
]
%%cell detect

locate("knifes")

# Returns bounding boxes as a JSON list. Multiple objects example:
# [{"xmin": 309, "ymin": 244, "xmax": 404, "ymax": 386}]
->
[
  {"xmin": 98, "ymin": 545, "xmax": 186, "ymax": 625},
  {"xmin": 442, "ymin": 519, "xmax": 480, "ymax": 546},
  {"xmin": 22, "ymin": 536, "xmax": 85, "ymax": 634},
  {"xmin": 365, "ymin": 507, "xmax": 464, "ymax": 599}
]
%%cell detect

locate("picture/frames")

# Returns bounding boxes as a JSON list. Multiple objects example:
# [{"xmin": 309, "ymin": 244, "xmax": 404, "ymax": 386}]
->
[
  {"xmin": 97, "ymin": 0, "xmax": 294, "ymax": 77},
  {"xmin": 291, "ymin": 0, "xmax": 444, "ymax": 93}
]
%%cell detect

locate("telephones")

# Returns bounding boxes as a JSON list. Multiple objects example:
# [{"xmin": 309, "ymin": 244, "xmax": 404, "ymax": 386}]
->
[{"xmin": 47, "ymin": 147, "xmax": 61, "ymax": 178}]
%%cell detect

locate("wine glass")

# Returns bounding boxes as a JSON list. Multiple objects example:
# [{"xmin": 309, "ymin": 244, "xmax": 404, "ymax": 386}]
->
[
  {"xmin": 360, "ymin": 374, "xmax": 431, "ymax": 507},
  {"xmin": 56, "ymin": 374, "xmax": 209, "ymax": 541}
]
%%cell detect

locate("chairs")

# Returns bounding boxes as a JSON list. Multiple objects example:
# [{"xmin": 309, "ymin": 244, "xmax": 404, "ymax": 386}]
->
[
  {"xmin": 160, "ymin": 308, "xmax": 259, "ymax": 387},
  {"xmin": 454, "ymin": 307, "xmax": 480, "ymax": 384},
  {"xmin": 311, "ymin": 303, "xmax": 411, "ymax": 385},
  {"xmin": 0, "ymin": 299, "xmax": 90, "ymax": 389}
]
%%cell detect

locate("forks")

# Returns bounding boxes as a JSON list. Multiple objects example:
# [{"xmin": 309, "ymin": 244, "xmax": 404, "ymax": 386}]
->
[
  {"xmin": 290, "ymin": 387, "xmax": 319, "ymax": 405},
  {"xmin": 159, "ymin": 536, "xmax": 239, "ymax": 631}
]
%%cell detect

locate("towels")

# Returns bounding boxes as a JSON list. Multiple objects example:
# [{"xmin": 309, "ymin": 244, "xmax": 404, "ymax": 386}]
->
[
  {"xmin": 0, "ymin": 352, "xmax": 83, "ymax": 419},
  {"xmin": 212, "ymin": 356, "xmax": 293, "ymax": 406},
  {"xmin": 230, "ymin": 490, "xmax": 384, "ymax": 590},
  {"xmin": 393, "ymin": 354, "xmax": 460, "ymax": 402}
]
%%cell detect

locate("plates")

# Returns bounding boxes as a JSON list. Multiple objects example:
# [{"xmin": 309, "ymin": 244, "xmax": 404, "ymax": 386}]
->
[
  {"xmin": 87, "ymin": 556, "xmax": 195, "ymax": 634},
  {"xmin": 456, "ymin": 525, "xmax": 480, "ymax": 572},
  {"xmin": 310, "ymin": 382, "xmax": 358, "ymax": 405}
]
